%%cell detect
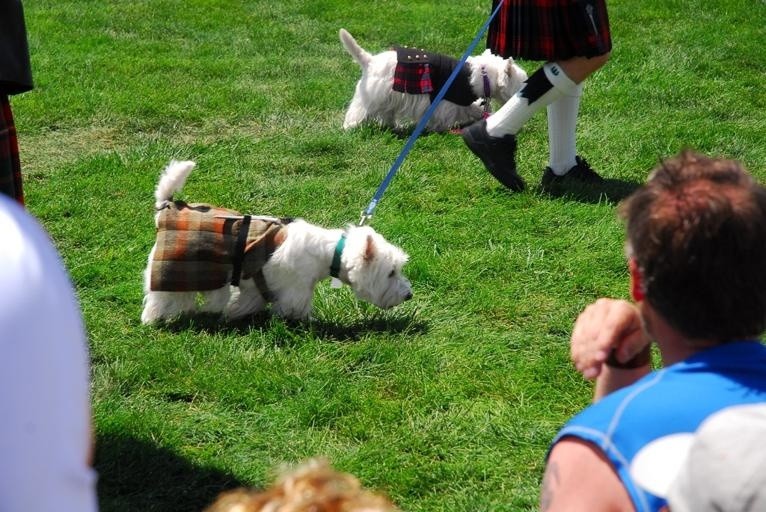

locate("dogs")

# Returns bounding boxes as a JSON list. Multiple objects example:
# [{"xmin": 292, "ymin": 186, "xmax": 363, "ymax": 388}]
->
[
  {"xmin": 338, "ymin": 28, "xmax": 528, "ymax": 134},
  {"xmin": 140, "ymin": 159, "xmax": 413, "ymax": 328}
]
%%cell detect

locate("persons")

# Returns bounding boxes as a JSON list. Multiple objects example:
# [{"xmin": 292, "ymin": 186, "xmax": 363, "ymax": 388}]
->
[
  {"xmin": 535, "ymin": 145, "xmax": 766, "ymax": 512},
  {"xmin": 624, "ymin": 401, "xmax": 766, "ymax": 512},
  {"xmin": 462, "ymin": 1, "xmax": 616, "ymax": 198}
]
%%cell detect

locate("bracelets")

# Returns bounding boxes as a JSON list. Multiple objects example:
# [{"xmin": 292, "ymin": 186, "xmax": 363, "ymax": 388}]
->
[{"xmin": 604, "ymin": 346, "xmax": 651, "ymax": 369}]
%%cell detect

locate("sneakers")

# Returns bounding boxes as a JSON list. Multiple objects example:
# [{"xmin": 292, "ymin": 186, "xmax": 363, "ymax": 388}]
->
[
  {"xmin": 462, "ymin": 119, "xmax": 525, "ymax": 194},
  {"xmin": 541, "ymin": 155, "xmax": 615, "ymax": 196}
]
